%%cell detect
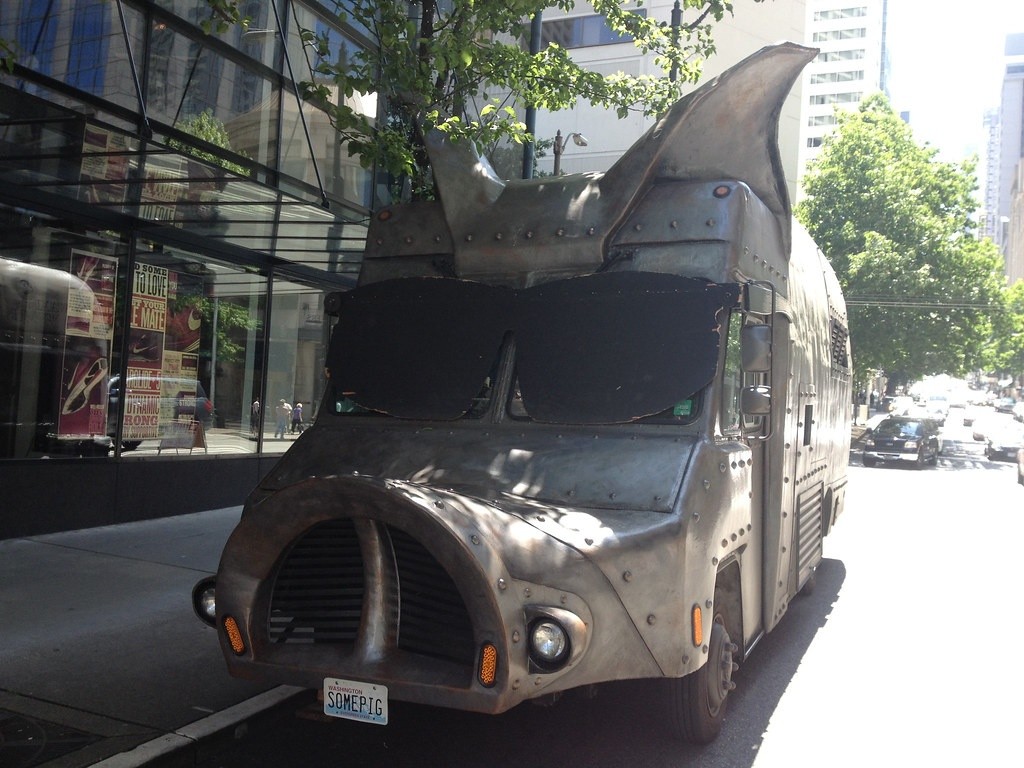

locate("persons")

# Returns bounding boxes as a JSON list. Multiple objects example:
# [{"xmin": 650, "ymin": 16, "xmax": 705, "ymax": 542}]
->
[
  {"xmin": 253, "ymin": 396, "xmax": 260, "ymax": 437},
  {"xmin": 274, "ymin": 399, "xmax": 303, "ymax": 440}
]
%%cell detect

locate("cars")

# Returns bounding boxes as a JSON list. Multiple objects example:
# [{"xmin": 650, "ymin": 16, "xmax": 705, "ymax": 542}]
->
[
  {"xmin": 964, "ymin": 406, "xmax": 1024, "ymax": 483},
  {"xmin": 889, "ymin": 376, "xmax": 969, "ymax": 425},
  {"xmin": 862, "ymin": 417, "xmax": 939, "ymax": 468},
  {"xmin": 972, "ymin": 390, "xmax": 1024, "ymax": 421}
]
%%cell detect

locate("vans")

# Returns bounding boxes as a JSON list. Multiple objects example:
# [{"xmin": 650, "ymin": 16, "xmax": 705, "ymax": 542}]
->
[{"xmin": 110, "ymin": 376, "xmax": 209, "ymax": 446}]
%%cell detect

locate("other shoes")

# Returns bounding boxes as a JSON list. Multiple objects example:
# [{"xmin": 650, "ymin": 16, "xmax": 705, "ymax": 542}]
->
[
  {"xmin": 274, "ymin": 435, "xmax": 277, "ymax": 437},
  {"xmin": 299, "ymin": 433, "xmax": 301, "ymax": 435},
  {"xmin": 291, "ymin": 431, "xmax": 294, "ymax": 434},
  {"xmin": 280, "ymin": 437, "xmax": 284, "ymax": 440}
]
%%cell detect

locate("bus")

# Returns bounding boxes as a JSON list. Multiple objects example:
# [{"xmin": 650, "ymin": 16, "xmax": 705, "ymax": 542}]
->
[
  {"xmin": 0, "ymin": 259, "xmax": 107, "ymax": 458},
  {"xmin": 193, "ymin": 42, "xmax": 853, "ymax": 744}
]
